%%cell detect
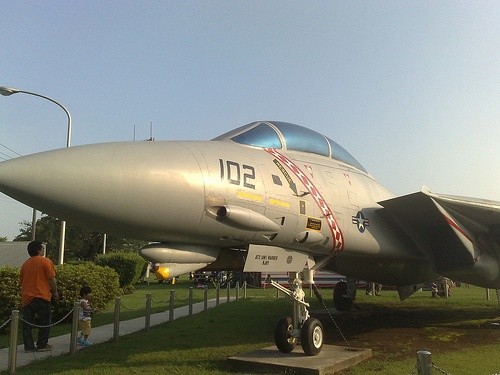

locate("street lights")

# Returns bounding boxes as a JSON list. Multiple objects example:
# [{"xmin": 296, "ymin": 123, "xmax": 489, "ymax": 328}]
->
[{"xmin": 0, "ymin": 87, "xmax": 73, "ymax": 265}]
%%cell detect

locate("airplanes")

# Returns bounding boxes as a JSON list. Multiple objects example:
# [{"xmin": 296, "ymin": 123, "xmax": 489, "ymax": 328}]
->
[{"xmin": 0, "ymin": 120, "xmax": 500, "ymax": 356}]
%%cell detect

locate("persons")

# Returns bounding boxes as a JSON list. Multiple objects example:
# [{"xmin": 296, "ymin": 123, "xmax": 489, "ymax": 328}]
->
[
  {"xmin": 20, "ymin": 240, "xmax": 59, "ymax": 352},
  {"xmin": 78, "ymin": 287, "xmax": 94, "ymax": 346}
]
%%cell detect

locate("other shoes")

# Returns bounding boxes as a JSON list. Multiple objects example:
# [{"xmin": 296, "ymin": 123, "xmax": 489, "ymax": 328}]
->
[
  {"xmin": 81, "ymin": 339, "xmax": 92, "ymax": 346},
  {"xmin": 77, "ymin": 337, "xmax": 83, "ymax": 344},
  {"xmin": 24, "ymin": 346, "xmax": 38, "ymax": 352},
  {"xmin": 37, "ymin": 344, "xmax": 53, "ymax": 351}
]
